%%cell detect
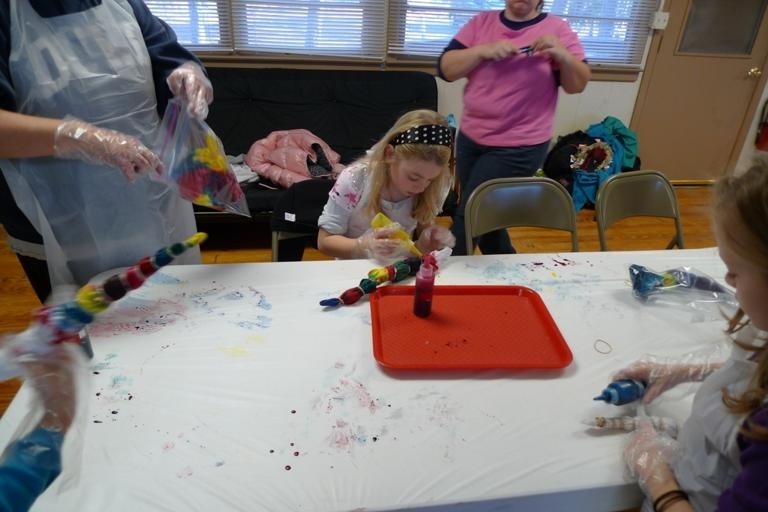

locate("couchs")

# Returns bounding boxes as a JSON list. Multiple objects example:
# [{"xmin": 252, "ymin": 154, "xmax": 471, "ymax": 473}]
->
[{"xmin": 183, "ymin": 68, "xmax": 444, "ymax": 255}]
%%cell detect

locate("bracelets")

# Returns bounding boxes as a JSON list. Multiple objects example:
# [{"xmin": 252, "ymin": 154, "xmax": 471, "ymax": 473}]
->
[{"xmin": 653, "ymin": 488, "xmax": 689, "ymax": 511}]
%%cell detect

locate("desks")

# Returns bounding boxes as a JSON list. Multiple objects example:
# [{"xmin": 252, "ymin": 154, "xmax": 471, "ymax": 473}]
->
[{"xmin": 0, "ymin": 248, "xmax": 763, "ymax": 510}]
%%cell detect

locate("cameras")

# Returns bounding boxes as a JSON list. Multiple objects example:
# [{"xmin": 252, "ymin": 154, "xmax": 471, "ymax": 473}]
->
[{"xmin": 518, "ymin": 44, "xmax": 534, "ymax": 56}]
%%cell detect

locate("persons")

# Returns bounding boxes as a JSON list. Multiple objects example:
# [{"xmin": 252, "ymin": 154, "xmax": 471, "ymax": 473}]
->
[
  {"xmin": 609, "ymin": 152, "xmax": 767, "ymax": 511},
  {"xmin": 314, "ymin": 108, "xmax": 458, "ymax": 258},
  {"xmin": 437, "ymin": 1, "xmax": 591, "ymax": 255},
  {"xmin": 1, "ymin": 334, "xmax": 81, "ymax": 511},
  {"xmin": 0, "ymin": 1, "xmax": 216, "ymax": 306}
]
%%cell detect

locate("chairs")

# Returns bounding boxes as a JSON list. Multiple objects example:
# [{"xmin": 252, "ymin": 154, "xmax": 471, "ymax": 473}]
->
[
  {"xmin": 462, "ymin": 175, "xmax": 581, "ymax": 257},
  {"xmin": 593, "ymin": 169, "xmax": 695, "ymax": 252},
  {"xmin": 271, "ymin": 171, "xmax": 337, "ymax": 264}
]
